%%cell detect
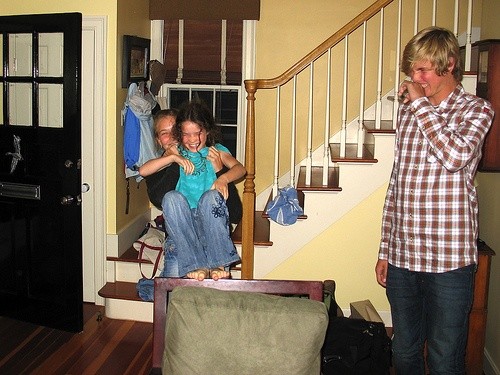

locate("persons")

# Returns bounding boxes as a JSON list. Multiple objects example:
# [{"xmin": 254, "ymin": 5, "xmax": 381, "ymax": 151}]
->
[
  {"xmin": 139, "ymin": 102, "xmax": 246, "ymax": 249},
  {"xmin": 376, "ymin": 26, "xmax": 495, "ymax": 375},
  {"xmin": 146, "ymin": 109, "xmax": 242, "ymax": 281}
]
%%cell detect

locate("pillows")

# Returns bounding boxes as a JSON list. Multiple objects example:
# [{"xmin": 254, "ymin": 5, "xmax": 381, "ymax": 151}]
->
[{"xmin": 162, "ymin": 285, "xmax": 329, "ymax": 374}]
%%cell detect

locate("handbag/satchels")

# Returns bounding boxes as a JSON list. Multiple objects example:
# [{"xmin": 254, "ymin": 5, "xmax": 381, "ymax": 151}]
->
[
  {"xmin": 321, "ymin": 317, "xmax": 392, "ymax": 375},
  {"xmin": 133, "ymin": 227, "xmax": 165, "ymax": 271}
]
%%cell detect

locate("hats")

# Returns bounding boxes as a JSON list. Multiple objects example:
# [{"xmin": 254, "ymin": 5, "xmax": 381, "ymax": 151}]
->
[{"xmin": 149, "ymin": 60, "xmax": 166, "ymax": 95}]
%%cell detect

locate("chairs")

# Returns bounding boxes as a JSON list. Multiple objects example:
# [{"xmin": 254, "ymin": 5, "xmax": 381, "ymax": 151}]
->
[{"xmin": 152, "ymin": 277, "xmax": 337, "ymax": 375}]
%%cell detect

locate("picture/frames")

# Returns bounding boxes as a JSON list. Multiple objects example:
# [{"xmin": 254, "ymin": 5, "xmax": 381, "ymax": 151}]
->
[{"xmin": 122, "ymin": 35, "xmax": 151, "ymax": 89}]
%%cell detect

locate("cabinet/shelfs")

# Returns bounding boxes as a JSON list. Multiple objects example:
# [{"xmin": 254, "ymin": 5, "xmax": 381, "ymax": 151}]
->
[
  {"xmin": 423, "ymin": 238, "xmax": 496, "ymax": 375},
  {"xmin": 458, "ymin": 39, "xmax": 500, "ymax": 173}
]
